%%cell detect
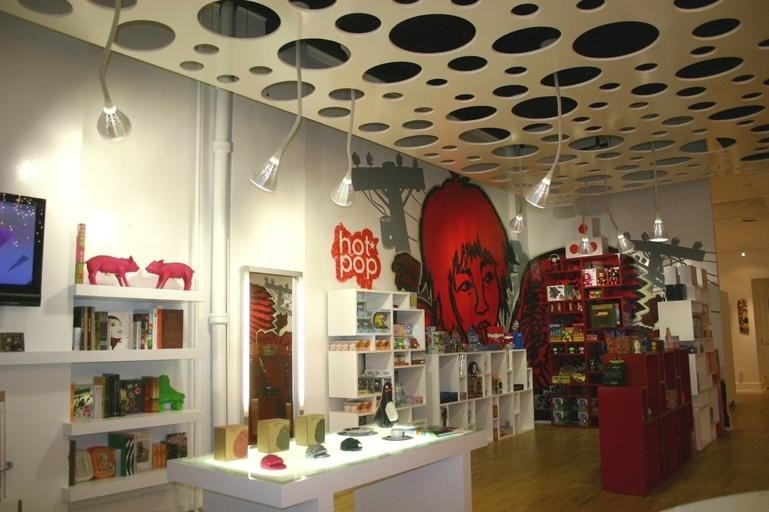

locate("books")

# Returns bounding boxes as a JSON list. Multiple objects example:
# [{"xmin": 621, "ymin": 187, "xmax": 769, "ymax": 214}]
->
[
  {"xmin": 399, "ymin": 423, "xmax": 465, "ymax": 438},
  {"xmin": 73, "ymin": 300, "xmax": 190, "ymax": 483}
]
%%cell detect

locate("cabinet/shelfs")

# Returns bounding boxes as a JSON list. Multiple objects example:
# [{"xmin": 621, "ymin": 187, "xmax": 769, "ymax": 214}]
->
[
  {"xmin": 62, "ymin": 284, "xmax": 206, "ymax": 503},
  {"xmin": 327, "ymin": 288, "xmax": 535, "ymax": 441}
]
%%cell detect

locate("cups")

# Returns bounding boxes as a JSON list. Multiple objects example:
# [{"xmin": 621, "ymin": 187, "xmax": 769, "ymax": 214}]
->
[{"xmin": 390, "ymin": 429, "xmax": 405, "ymax": 438}]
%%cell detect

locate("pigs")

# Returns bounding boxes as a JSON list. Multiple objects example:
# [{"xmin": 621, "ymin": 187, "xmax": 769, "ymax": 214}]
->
[
  {"xmin": 85, "ymin": 255, "xmax": 140, "ymax": 287},
  {"xmin": 145, "ymin": 259, "xmax": 195, "ymax": 291}
]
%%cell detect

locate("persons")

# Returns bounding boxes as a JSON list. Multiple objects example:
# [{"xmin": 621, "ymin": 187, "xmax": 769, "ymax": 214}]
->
[{"xmin": 391, "ymin": 173, "xmax": 517, "ymax": 344}]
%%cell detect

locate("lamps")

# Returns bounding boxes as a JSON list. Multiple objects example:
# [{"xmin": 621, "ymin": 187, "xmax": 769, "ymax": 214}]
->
[
  {"xmin": 250, "ymin": 11, "xmax": 303, "ymax": 195},
  {"xmin": 96, "ymin": 1, "xmax": 133, "ymax": 140},
  {"xmin": 507, "ymin": 73, "xmax": 562, "ymax": 233},
  {"xmin": 577, "ymin": 143, "xmax": 670, "ymax": 254},
  {"xmin": 330, "ymin": 89, "xmax": 356, "ymax": 208}
]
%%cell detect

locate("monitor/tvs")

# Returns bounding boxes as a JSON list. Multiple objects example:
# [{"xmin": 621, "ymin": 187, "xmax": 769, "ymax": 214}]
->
[{"xmin": 0, "ymin": 191, "xmax": 46, "ymax": 307}]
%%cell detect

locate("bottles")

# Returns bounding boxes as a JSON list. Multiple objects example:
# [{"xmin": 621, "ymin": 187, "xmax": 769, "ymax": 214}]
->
[
  {"xmin": 72, "ymin": 321, "xmax": 81, "ymax": 350},
  {"xmin": 376, "ymin": 382, "xmax": 396, "ymax": 428}
]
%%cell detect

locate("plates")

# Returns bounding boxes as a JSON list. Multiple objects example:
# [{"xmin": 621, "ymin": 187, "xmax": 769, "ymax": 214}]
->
[
  {"xmin": 384, "ymin": 435, "xmax": 410, "ymax": 440},
  {"xmin": 345, "ymin": 426, "xmax": 374, "ymax": 435}
]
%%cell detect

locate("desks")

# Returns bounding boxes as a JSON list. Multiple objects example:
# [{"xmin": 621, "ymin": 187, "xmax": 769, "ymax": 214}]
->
[{"xmin": 167, "ymin": 429, "xmax": 489, "ymax": 512}]
